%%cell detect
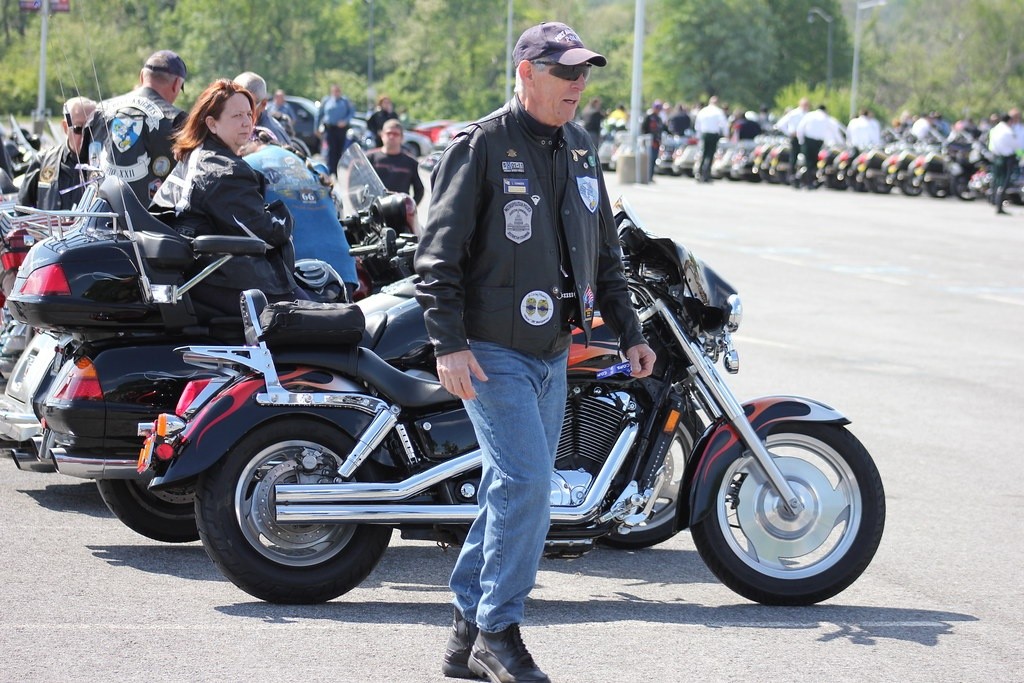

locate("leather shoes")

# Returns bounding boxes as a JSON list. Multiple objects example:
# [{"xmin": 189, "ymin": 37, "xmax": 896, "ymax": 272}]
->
[
  {"xmin": 468, "ymin": 623, "xmax": 552, "ymax": 683},
  {"xmin": 442, "ymin": 606, "xmax": 478, "ymax": 679}
]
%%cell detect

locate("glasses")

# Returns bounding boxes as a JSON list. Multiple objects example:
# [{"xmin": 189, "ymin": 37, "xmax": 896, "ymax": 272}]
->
[
  {"xmin": 549, "ymin": 64, "xmax": 592, "ymax": 81},
  {"xmin": 383, "ymin": 132, "xmax": 401, "ymax": 136},
  {"xmin": 69, "ymin": 126, "xmax": 82, "ymax": 135}
]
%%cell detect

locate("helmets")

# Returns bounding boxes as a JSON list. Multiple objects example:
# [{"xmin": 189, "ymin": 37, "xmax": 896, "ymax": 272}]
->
[{"xmin": 294, "ymin": 258, "xmax": 348, "ymax": 303}]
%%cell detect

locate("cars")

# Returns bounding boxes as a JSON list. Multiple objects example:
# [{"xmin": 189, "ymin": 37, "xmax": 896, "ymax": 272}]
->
[{"xmin": 285, "ymin": 97, "xmax": 472, "ymax": 158}]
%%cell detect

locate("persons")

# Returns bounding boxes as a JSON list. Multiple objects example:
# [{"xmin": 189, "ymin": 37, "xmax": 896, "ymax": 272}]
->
[
  {"xmin": 80, "ymin": 48, "xmax": 190, "ymax": 212},
  {"xmin": 149, "ymin": 79, "xmax": 316, "ymax": 317},
  {"xmin": 316, "ymin": 84, "xmax": 353, "ymax": 183},
  {"xmin": 987, "ymin": 114, "xmax": 1017, "ymax": 215},
  {"xmin": 413, "ymin": 20, "xmax": 659, "ymax": 683},
  {"xmin": 17, "ymin": 48, "xmax": 360, "ymax": 303},
  {"xmin": 16, "ymin": 95, "xmax": 97, "ymax": 215},
  {"xmin": 694, "ymin": 95, "xmax": 729, "ymax": 183},
  {"xmin": 579, "ymin": 95, "xmax": 1024, "ymax": 215},
  {"xmin": 350, "ymin": 93, "xmax": 425, "ymax": 216}
]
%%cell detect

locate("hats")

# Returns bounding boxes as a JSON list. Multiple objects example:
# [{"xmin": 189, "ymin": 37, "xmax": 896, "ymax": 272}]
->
[
  {"xmin": 144, "ymin": 50, "xmax": 187, "ymax": 93},
  {"xmin": 512, "ymin": 22, "xmax": 607, "ymax": 67}
]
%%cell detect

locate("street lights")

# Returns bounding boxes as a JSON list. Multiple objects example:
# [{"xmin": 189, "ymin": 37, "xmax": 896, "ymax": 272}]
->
[
  {"xmin": 807, "ymin": 8, "xmax": 834, "ymax": 99},
  {"xmin": 847, "ymin": 0, "xmax": 888, "ymax": 118}
]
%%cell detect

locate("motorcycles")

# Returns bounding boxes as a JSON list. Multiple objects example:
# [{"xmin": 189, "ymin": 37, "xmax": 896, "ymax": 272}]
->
[
  {"xmin": 135, "ymin": 201, "xmax": 884, "ymax": 604},
  {"xmin": 0, "ymin": 116, "xmax": 709, "ymax": 551},
  {"xmin": 598, "ymin": 112, "xmax": 1024, "ymax": 206}
]
